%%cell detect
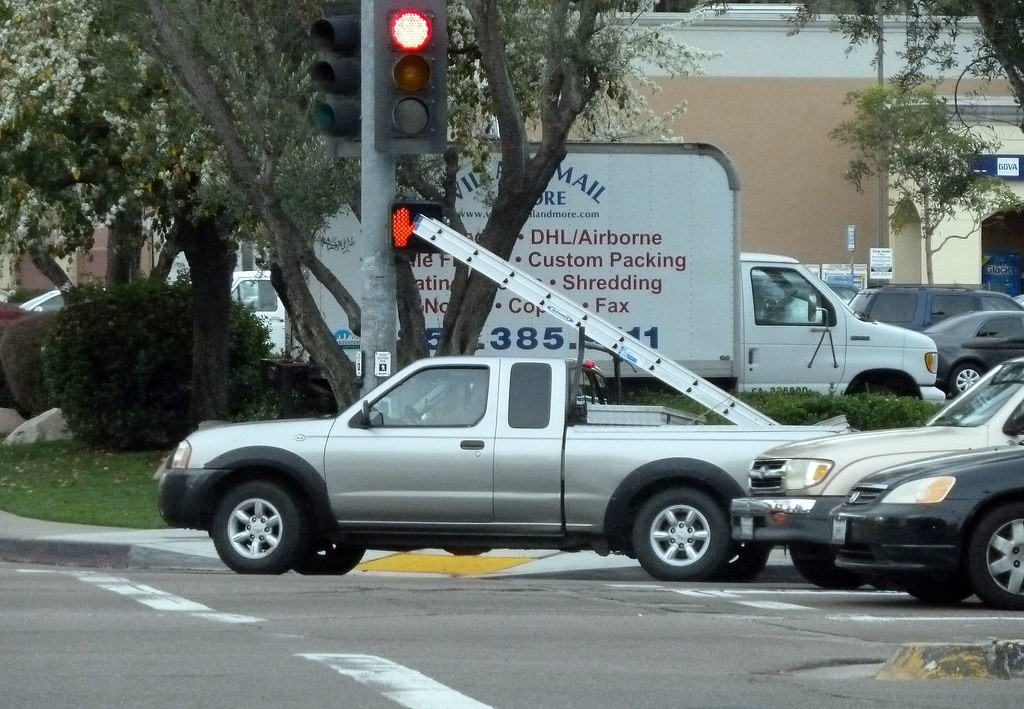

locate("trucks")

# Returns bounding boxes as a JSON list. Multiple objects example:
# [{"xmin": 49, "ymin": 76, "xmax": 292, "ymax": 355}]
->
[{"xmin": 282, "ymin": 140, "xmax": 947, "ymax": 406}]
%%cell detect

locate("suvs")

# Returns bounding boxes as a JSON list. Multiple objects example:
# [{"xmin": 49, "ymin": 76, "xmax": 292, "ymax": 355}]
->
[{"xmin": 847, "ymin": 283, "xmax": 1024, "ymax": 336}]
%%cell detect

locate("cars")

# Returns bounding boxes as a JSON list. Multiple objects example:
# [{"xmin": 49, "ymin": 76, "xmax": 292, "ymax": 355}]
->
[
  {"xmin": 922, "ymin": 311, "xmax": 1024, "ymax": 383},
  {"xmin": 828, "ymin": 444, "xmax": 1024, "ymax": 611},
  {"xmin": 21, "ymin": 289, "xmax": 65, "ymax": 315},
  {"xmin": 731, "ymin": 358, "xmax": 1024, "ymax": 594}
]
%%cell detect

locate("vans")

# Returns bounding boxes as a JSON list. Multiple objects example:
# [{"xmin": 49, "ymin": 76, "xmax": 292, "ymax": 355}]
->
[{"xmin": 229, "ymin": 271, "xmax": 287, "ymax": 357}]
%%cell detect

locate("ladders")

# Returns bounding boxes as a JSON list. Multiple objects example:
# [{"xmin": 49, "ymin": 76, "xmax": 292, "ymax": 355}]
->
[{"xmin": 411, "ymin": 215, "xmax": 781, "ymax": 426}]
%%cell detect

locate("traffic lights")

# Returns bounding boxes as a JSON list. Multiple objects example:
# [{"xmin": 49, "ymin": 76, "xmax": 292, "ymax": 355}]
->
[
  {"xmin": 389, "ymin": 202, "xmax": 447, "ymax": 254},
  {"xmin": 309, "ymin": 1, "xmax": 364, "ymax": 157},
  {"xmin": 373, "ymin": 0, "xmax": 451, "ymax": 158}
]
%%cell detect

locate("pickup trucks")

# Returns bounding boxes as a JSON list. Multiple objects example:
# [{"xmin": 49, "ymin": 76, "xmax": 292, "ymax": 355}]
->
[{"xmin": 152, "ymin": 356, "xmax": 855, "ymax": 588}]
